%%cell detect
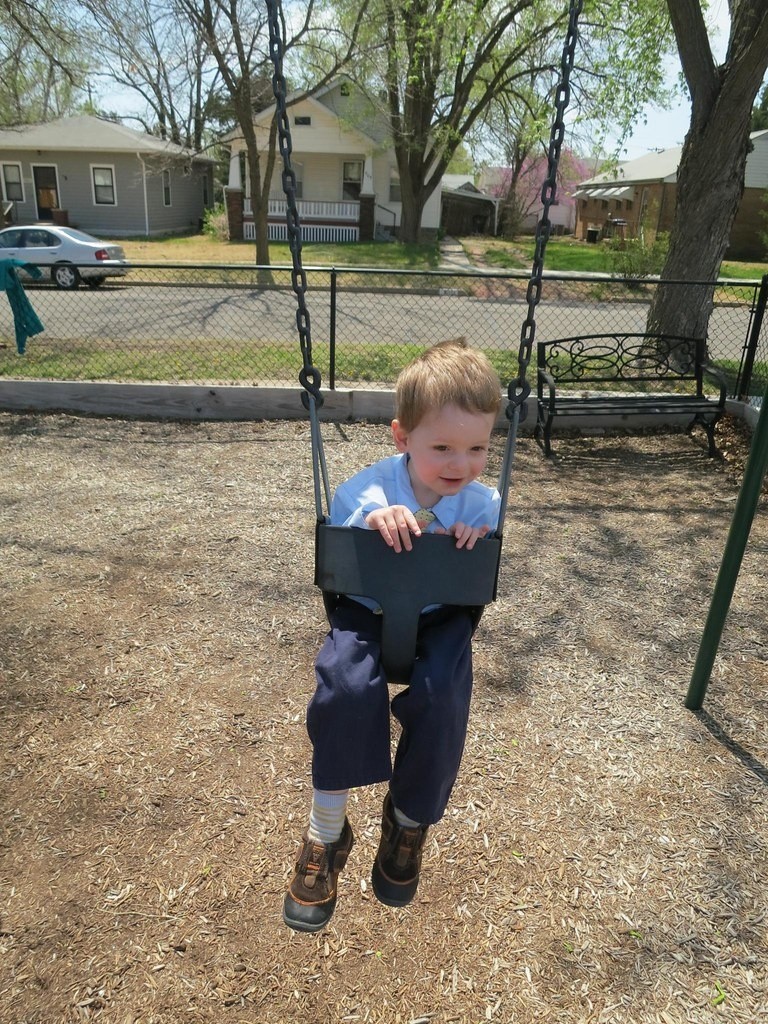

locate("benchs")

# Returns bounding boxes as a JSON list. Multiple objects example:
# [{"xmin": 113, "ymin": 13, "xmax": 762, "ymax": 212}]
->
[{"xmin": 534, "ymin": 333, "xmax": 726, "ymax": 456}]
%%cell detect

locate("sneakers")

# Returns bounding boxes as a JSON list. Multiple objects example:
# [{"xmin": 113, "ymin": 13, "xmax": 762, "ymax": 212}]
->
[
  {"xmin": 371, "ymin": 791, "xmax": 429, "ymax": 907},
  {"xmin": 282, "ymin": 815, "xmax": 353, "ymax": 932}
]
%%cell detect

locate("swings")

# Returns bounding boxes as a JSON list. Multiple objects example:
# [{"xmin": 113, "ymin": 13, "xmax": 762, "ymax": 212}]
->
[{"xmin": 266, "ymin": 0, "xmax": 587, "ymax": 686}]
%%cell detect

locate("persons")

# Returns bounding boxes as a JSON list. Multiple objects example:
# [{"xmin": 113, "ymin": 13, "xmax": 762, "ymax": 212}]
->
[{"xmin": 282, "ymin": 337, "xmax": 502, "ymax": 933}]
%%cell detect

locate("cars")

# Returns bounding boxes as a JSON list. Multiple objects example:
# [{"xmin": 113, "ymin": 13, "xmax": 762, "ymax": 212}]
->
[{"xmin": 0, "ymin": 224, "xmax": 128, "ymax": 291}]
